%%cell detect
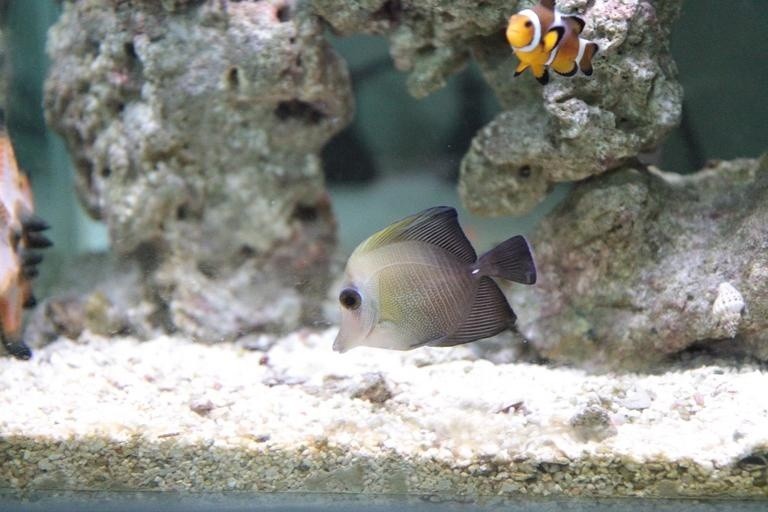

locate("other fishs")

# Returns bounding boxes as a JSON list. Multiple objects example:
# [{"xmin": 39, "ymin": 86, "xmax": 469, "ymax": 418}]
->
[
  {"xmin": 505, "ymin": 0, "xmax": 598, "ymax": 85},
  {"xmin": 331, "ymin": 205, "xmax": 538, "ymax": 356},
  {"xmin": 0, "ymin": 108, "xmax": 53, "ymax": 362}
]
